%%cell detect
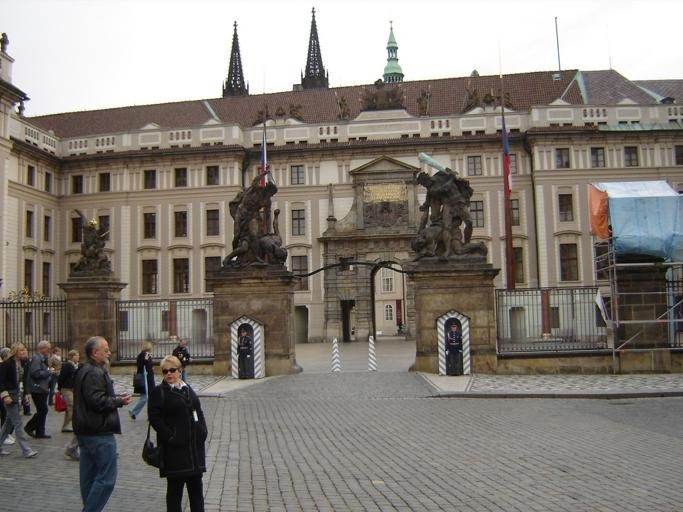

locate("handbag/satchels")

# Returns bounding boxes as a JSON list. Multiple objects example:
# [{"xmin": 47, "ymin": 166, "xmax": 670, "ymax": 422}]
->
[
  {"xmin": 134, "ymin": 373, "xmax": 146, "ymax": 394},
  {"xmin": 142, "ymin": 439, "xmax": 175, "ymax": 468},
  {"xmin": 55, "ymin": 392, "xmax": 67, "ymax": 412}
]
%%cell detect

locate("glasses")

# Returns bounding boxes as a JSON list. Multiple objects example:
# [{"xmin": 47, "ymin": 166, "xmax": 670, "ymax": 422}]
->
[{"xmin": 162, "ymin": 367, "xmax": 179, "ymax": 375}]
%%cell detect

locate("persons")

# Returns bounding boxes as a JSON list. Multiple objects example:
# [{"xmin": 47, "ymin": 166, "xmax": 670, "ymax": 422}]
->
[
  {"xmin": 146, "ymin": 352, "xmax": 209, "ymax": 512},
  {"xmin": 412, "ymin": 164, "xmax": 476, "ymax": 263},
  {"xmin": 171, "ymin": 337, "xmax": 193, "ymax": 383},
  {"xmin": 414, "ymin": 88, "xmax": 432, "ymax": 117},
  {"xmin": 444, "ymin": 320, "xmax": 463, "ymax": 377},
  {"xmin": 71, "ymin": 335, "xmax": 133, "ymax": 512},
  {"xmin": 228, "ymin": 168, "xmax": 277, "ymax": 261},
  {"xmin": 0, "ymin": 338, "xmax": 82, "ymax": 463},
  {"xmin": 404, "ymin": 213, "xmax": 487, "ymax": 265},
  {"xmin": 222, "ymin": 206, "xmax": 288, "ymax": 269},
  {"xmin": 333, "ymin": 96, "xmax": 352, "ymax": 121},
  {"xmin": 236, "ymin": 328, "xmax": 253, "ymax": 377},
  {"xmin": 127, "ymin": 339, "xmax": 158, "ymax": 423}
]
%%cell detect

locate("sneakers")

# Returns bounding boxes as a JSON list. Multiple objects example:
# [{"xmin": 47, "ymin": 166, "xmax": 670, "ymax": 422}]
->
[
  {"xmin": 0, "ymin": 426, "xmax": 52, "ymax": 459},
  {"xmin": 64, "ymin": 446, "xmax": 80, "ymax": 460},
  {"xmin": 131, "ymin": 415, "xmax": 136, "ymax": 419}
]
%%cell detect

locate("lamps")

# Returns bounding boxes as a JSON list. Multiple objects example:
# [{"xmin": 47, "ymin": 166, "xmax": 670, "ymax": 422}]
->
[{"xmin": 326, "ymin": 215, "xmax": 338, "ymax": 229}]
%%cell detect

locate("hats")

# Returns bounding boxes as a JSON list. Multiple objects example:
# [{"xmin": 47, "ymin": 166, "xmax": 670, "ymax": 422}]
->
[{"xmin": 1, "ymin": 348, "xmax": 10, "ymax": 360}]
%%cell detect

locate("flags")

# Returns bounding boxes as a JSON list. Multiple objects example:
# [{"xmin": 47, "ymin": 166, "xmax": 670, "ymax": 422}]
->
[
  {"xmin": 498, "ymin": 73, "xmax": 515, "ymax": 200},
  {"xmin": 259, "ymin": 95, "xmax": 273, "ymax": 191}
]
